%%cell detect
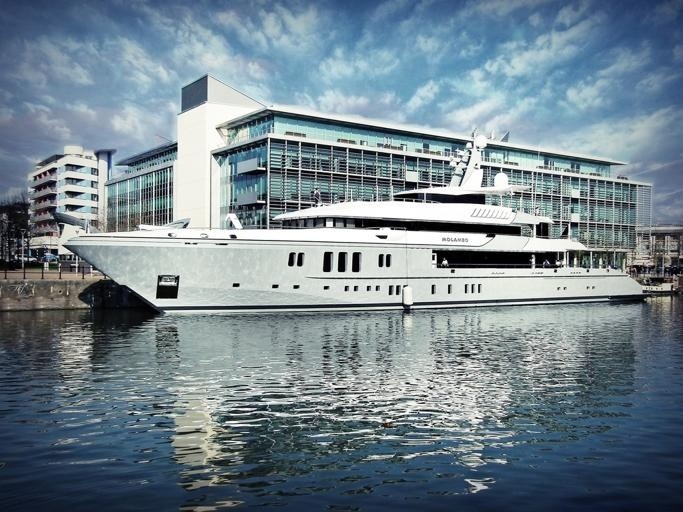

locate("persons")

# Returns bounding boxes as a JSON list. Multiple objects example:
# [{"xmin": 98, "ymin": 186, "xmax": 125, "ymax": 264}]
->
[
  {"xmin": 542, "ymin": 257, "xmax": 550, "ymax": 268},
  {"xmin": 440, "ymin": 256, "xmax": 449, "ymax": 268},
  {"xmin": 553, "ymin": 259, "xmax": 561, "ymax": 268},
  {"xmin": 641, "ymin": 279, "xmax": 650, "ymax": 286},
  {"xmin": 313, "ymin": 188, "xmax": 320, "ymax": 207},
  {"xmin": 43, "ymin": 256, "xmax": 49, "ymax": 271},
  {"xmin": 605, "ymin": 262, "xmax": 611, "ymax": 269}
]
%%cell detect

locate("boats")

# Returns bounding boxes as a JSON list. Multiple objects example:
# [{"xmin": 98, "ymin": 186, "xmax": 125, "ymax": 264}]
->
[{"xmin": 62, "ymin": 135, "xmax": 649, "ymax": 315}]
